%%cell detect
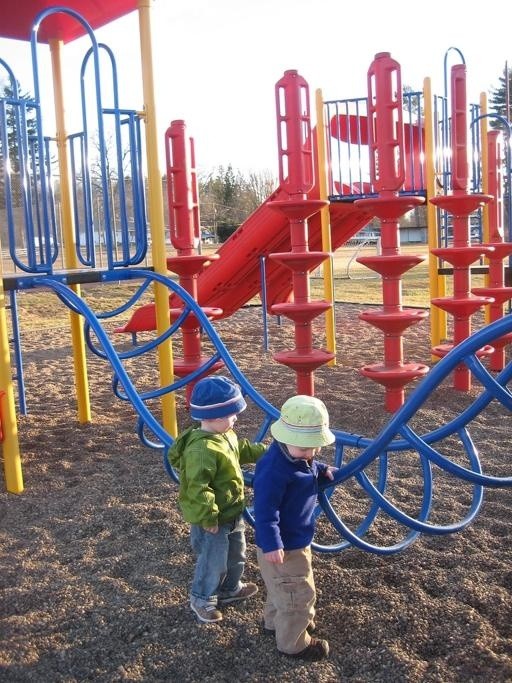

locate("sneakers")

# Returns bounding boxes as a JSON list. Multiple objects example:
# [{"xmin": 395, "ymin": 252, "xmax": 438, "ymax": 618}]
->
[
  {"xmin": 218, "ymin": 582, "xmax": 259, "ymax": 606},
  {"xmin": 190, "ymin": 602, "xmax": 222, "ymax": 622},
  {"xmin": 264, "ymin": 620, "xmax": 317, "ymax": 636},
  {"xmin": 278, "ymin": 637, "xmax": 330, "ymax": 662}
]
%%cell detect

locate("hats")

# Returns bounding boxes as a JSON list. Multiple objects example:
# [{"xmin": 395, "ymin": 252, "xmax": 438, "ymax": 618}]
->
[
  {"xmin": 270, "ymin": 394, "xmax": 336, "ymax": 448},
  {"xmin": 189, "ymin": 374, "xmax": 247, "ymax": 421}
]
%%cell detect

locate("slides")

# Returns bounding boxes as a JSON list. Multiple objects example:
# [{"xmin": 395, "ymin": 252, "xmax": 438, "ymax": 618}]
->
[{"xmin": 114, "ymin": 176, "xmax": 404, "ymax": 335}]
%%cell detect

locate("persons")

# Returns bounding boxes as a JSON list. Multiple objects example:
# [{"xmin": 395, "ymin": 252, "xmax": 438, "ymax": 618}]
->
[
  {"xmin": 251, "ymin": 394, "xmax": 340, "ymax": 662},
  {"xmin": 167, "ymin": 374, "xmax": 269, "ymax": 624}
]
